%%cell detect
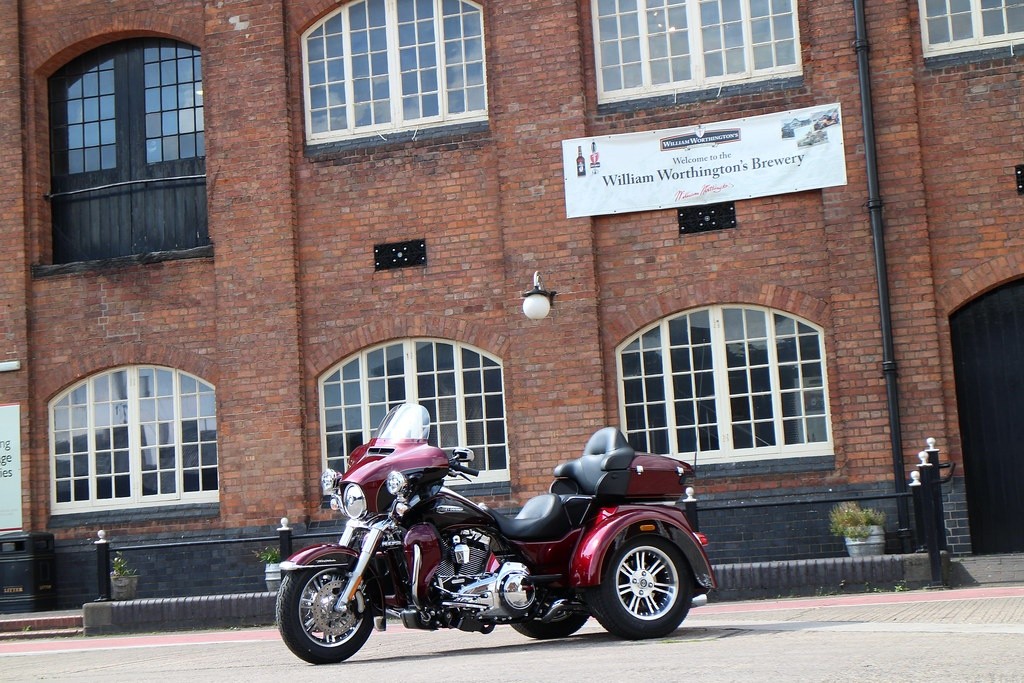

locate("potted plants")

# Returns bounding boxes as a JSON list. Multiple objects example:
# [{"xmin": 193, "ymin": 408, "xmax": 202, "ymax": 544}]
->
[
  {"xmin": 252, "ymin": 546, "xmax": 281, "ymax": 591},
  {"xmin": 828, "ymin": 500, "xmax": 887, "ymax": 557},
  {"xmin": 108, "ymin": 551, "xmax": 140, "ymax": 601}
]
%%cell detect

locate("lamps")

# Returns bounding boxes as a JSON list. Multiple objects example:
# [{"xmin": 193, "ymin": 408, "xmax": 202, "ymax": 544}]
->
[{"xmin": 522, "ymin": 271, "xmax": 556, "ymax": 321}]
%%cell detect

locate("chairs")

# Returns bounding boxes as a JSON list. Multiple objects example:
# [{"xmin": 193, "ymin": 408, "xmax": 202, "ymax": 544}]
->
[{"xmin": 549, "ymin": 427, "xmax": 635, "ymax": 527}]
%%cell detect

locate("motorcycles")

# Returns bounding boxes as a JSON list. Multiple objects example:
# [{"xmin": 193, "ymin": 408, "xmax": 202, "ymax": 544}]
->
[{"xmin": 274, "ymin": 403, "xmax": 718, "ymax": 667}]
